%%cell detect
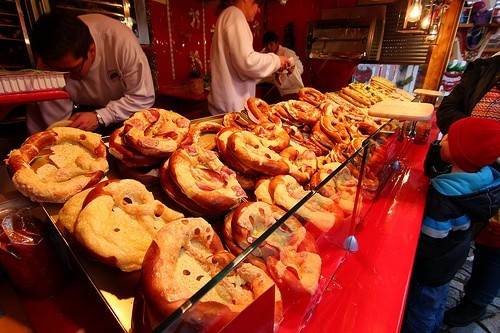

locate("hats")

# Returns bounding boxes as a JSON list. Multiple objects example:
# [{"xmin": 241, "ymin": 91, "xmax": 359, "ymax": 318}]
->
[{"xmin": 448, "ymin": 117, "xmax": 500, "ymax": 173}]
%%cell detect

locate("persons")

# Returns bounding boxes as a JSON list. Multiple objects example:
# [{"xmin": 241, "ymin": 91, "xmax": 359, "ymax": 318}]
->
[
  {"xmin": 26, "ymin": 12, "xmax": 156, "ymax": 136},
  {"xmin": 405, "ymin": 117, "xmax": 500, "ymax": 333},
  {"xmin": 256, "ymin": 31, "xmax": 304, "ymax": 101},
  {"xmin": 434, "ymin": 54, "xmax": 500, "ymax": 327},
  {"xmin": 207, "ymin": 0, "xmax": 292, "ymax": 116}
]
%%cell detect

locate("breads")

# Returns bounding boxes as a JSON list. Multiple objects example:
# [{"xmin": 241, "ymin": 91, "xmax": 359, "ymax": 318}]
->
[{"xmin": 4, "ymin": 76, "xmax": 394, "ymax": 333}]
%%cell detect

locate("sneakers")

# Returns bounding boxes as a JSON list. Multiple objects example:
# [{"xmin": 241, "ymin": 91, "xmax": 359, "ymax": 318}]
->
[{"xmin": 443, "ymin": 297, "xmax": 486, "ymax": 328}]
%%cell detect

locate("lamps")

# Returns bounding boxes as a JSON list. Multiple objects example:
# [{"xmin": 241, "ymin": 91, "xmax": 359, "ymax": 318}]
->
[{"xmin": 404, "ymin": 0, "xmax": 423, "ymax": 22}]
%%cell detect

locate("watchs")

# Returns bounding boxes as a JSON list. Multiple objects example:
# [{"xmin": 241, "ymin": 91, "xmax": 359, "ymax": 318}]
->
[{"xmin": 93, "ymin": 111, "xmax": 104, "ymax": 126}]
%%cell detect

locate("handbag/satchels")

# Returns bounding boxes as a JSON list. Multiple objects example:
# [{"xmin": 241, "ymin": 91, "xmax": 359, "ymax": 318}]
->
[{"xmin": 273, "ymin": 56, "xmax": 305, "ymax": 96}]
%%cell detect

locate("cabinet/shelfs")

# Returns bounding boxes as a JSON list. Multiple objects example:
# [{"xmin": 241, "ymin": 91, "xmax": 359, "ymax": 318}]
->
[{"xmin": 432, "ymin": 0, "xmax": 500, "ymax": 106}]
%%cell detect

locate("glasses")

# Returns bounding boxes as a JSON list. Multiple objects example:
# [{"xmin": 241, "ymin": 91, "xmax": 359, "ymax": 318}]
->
[{"xmin": 63, "ymin": 57, "xmax": 86, "ymax": 76}]
